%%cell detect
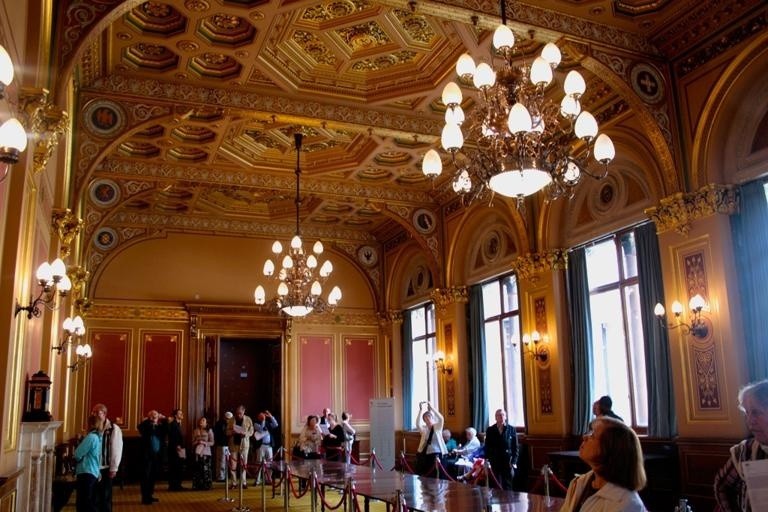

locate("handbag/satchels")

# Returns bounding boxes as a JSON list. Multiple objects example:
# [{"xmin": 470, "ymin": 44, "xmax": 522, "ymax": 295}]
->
[{"xmin": 292, "ymin": 441, "xmax": 306, "ymax": 459}]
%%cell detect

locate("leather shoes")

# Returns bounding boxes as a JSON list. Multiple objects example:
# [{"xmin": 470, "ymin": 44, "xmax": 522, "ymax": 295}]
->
[
  {"xmin": 141, "ymin": 496, "xmax": 152, "ymax": 505},
  {"xmin": 152, "ymin": 498, "xmax": 159, "ymax": 502},
  {"xmin": 168, "ymin": 480, "xmax": 274, "ymax": 493}
]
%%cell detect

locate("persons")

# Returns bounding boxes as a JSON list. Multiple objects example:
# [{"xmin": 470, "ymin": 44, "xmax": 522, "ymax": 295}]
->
[
  {"xmin": 485, "ymin": 409, "xmax": 519, "ymax": 491},
  {"xmin": 558, "ymin": 415, "xmax": 648, "ymax": 512},
  {"xmin": 600, "ymin": 396, "xmax": 624, "ymax": 421},
  {"xmin": 443, "ymin": 427, "xmax": 486, "ymax": 481},
  {"xmin": 588, "ymin": 400, "xmax": 602, "ymax": 433},
  {"xmin": 415, "ymin": 402, "xmax": 449, "ymax": 479},
  {"xmin": 137, "ymin": 405, "xmax": 279, "ymax": 506},
  {"xmin": 74, "ymin": 416, "xmax": 103, "ymax": 512},
  {"xmin": 295, "ymin": 407, "xmax": 357, "ymax": 489},
  {"xmin": 91, "ymin": 403, "xmax": 124, "ymax": 512},
  {"xmin": 712, "ymin": 378, "xmax": 768, "ymax": 512}
]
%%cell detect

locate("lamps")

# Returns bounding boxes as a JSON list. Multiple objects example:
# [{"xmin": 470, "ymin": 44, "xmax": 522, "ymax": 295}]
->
[
  {"xmin": 511, "ymin": 331, "xmax": 548, "ymax": 362},
  {"xmin": 15, "ymin": 257, "xmax": 72, "ymax": 320},
  {"xmin": 421, "ymin": 0, "xmax": 615, "ymax": 210},
  {"xmin": 1, "ymin": 46, "xmax": 27, "ymax": 182},
  {"xmin": 654, "ymin": 293, "xmax": 707, "ymax": 340},
  {"xmin": 253, "ymin": 126, "xmax": 342, "ymax": 319},
  {"xmin": 427, "ymin": 350, "xmax": 452, "ymax": 374},
  {"xmin": 52, "ymin": 315, "xmax": 85, "ymax": 354},
  {"xmin": 65, "ymin": 344, "xmax": 94, "ymax": 372}
]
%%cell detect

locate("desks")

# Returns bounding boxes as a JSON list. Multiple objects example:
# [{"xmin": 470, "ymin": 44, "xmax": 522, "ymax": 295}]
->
[{"xmin": 547, "ymin": 451, "xmax": 666, "ymax": 512}]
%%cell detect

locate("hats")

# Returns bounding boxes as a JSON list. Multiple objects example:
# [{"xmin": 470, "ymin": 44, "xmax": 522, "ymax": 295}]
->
[{"xmin": 225, "ymin": 411, "xmax": 233, "ymax": 418}]
